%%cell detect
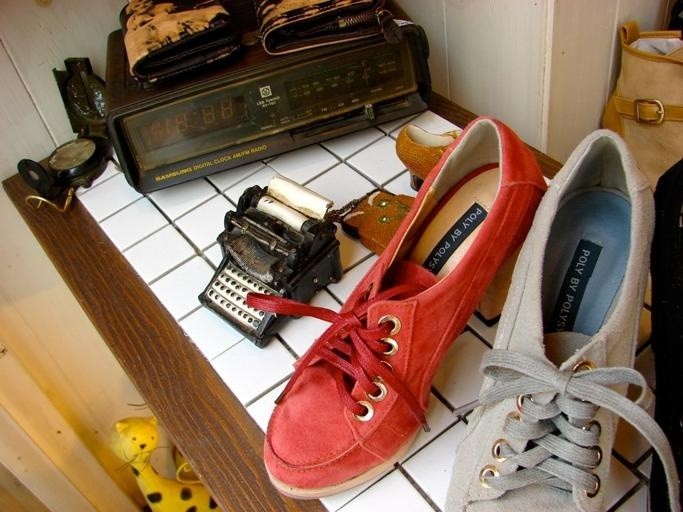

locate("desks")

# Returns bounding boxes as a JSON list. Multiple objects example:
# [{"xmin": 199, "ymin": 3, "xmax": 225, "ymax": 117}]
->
[{"xmin": 1, "ymin": 93, "xmax": 656, "ymax": 512}]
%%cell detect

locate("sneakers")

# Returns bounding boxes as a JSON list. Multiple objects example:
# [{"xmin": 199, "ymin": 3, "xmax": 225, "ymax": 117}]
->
[
  {"xmin": 645, "ymin": 156, "xmax": 681, "ymax": 510},
  {"xmin": 442, "ymin": 128, "xmax": 659, "ymax": 512},
  {"xmin": 260, "ymin": 114, "xmax": 549, "ymax": 500}
]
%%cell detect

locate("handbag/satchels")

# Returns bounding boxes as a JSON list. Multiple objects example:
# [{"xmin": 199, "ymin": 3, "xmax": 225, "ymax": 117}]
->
[
  {"xmin": 254, "ymin": 2, "xmax": 386, "ymax": 58},
  {"xmin": 599, "ymin": 22, "xmax": 682, "ymax": 193},
  {"xmin": 117, "ymin": 1, "xmax": 240, "ymax": 83}
]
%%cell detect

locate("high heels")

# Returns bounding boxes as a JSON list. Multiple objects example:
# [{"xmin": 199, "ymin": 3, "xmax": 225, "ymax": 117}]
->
[{"xmin": 393, "ymin": 121, "xmax": 460, "ymax": 192}]
